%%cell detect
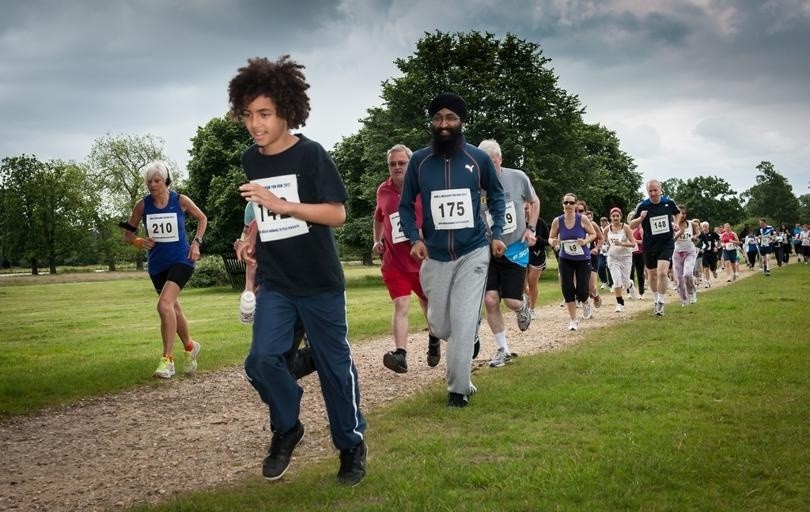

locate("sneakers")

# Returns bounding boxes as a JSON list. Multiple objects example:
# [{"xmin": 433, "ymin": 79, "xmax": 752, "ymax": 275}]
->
[
  {"xmin": 705, "ymin": 281, "xmax": 710, "ymax": 288},
  {"xmin": 569, "ymin": 321, "xmax": 580, "ymax": 331},
  {"xmin": 336, "ymin": 441, "xmax": 369, "ymax": 490},
  {"xmin": 153, "ymin": 358, "xmax": 175, "ymax": 379},
  {"xmin": 515, "ymin": 294, "xmax": 532, "ymax": 332},
  {"xmin": 383, "ymin": 351, "xmax": 408, "ymax": 375},
  {"xmin": 616, "ymin": 305, "xmax": 625, "ymax": 313},
  {"xmin": 473, "ymin": 318, "xmax": 482, "ymax": 360},
  {"xmin": 712, "ymin": 271, "xmax": 718, "ymax": 278},
  {"xmin": 490, "ymin": 349, "xmax": 512, "ymax": 368},
  {"xmin": 656, "ymin": 306, "xmax": 665, "ymax": 316},
  {"xmin": 260, "ymin": 418, "xmax": 305, "ymax": 481},
  {"xmin": 591, "ymin": 295, "xmax": 603, "ymax": 308},
  {"xmin": 182, "ymin": 340, "xmax": 201, "ymax": 377},
  {"xmin": 445, "ymin": 393, "xmax": 468, "ymax": 408},
  {"xmin": 582, "ymin": 297, "xmax": 591, "ymax": 320},
  {"xmin": 427, "ymin": 341, "xmax": 441, "ymax": 367},
  {"xmin": 529, "ymin": 311, "xmax": 538, "ymax": 320},
  {"xmin": 681, "ymin": 303, "xmax": 687, "ymax": 308},
  {"xmin": 600, "ymin": 283, "xmax": 608, "ymax": 291},
  {"xmin": 629, "ymin": 281, "xmax": 638, "ymax": 300},
  {"xmin": 690, "ymin": 294, "xmax": 697, "ymax": 304}
]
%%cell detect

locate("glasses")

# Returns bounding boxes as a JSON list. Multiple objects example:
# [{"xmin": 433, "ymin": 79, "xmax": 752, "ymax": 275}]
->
[
  {"xmin": 575, "ymin": 210, "xmax": 583, "ymax": 213},
  {"xmin": 563, "ymin": 200, "xmax": 576, "ymax": 205},
  {"xmin": 432, "ymin": 116, "xmax": 459, "ymax": 124},
  {"xmin": 387, "ymin": 161, "xmax": 408, "ymax": 168}
]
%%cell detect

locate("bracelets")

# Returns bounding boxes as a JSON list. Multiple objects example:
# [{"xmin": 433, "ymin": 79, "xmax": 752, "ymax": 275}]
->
[{"xmin": 527, "ymin": 225, "xmax": 538, "ymax": 232}]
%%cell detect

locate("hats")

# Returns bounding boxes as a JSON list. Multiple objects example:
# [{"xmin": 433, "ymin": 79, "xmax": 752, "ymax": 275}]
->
[
  {"xmin": 428, "ymin": 93, "xmax": 468, "ymax": 122},
  {"xmin": 609, "ymin": 206, "xmax": 622, "ymax": 215}
]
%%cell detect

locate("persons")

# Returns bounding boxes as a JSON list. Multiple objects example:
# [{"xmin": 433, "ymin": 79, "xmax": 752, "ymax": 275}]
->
[
  {"xmin": 477, "ymin": 139, "xmax": 540, "ymax": 368},
  {"xmin": 372, "ymin": 144, "xmax": 441, "ymax": 374},
  {"xmin": 757, "ymin": 218, "xmax": 777, "ymax": 275},
  {"xmin": 800, "ymin": 225, "xmax": 810, "ymax": 263},
  {"xmin": 548, "ymin": 193, "xmax": 596, "ymax": 331},
  {"xmin": 597, "ymin": 217, "xmax": 614, "ymax": 293},
  {"xmin": 791, "ymin": 223, "xmax": 802, "ymax": 261},
  {"xmin": 522, "ymin": 198, "xmax": 549, "ymax": 322},
  {"xmin": 745, "ymin": 229, "xmax": 757, "ymax": 271},
  {"xmin": 636, "ymin": 203, "xmax": 649, "ymax": 290},
  {"xmin": 773, "ymin": 227, "xmax": 785, "ymax": 268},
  {"xmin": 603, "ymin": 207, "xmax": 636, "ymax": 313},
  {"xmin": 629, "ymin": 180, "xmax": 682, "ymax": 317},
  {"xmin": 721, "ymin": 223, "xmax": 740, "ymax": 282},
  {"xmin": 398, "ymin": 93, "xmax": 508, "ymax": 408},
  {"xmin": 672, "ymin": 205, "xmax": 701, "ymax": 307},
  {"xmin": 227, "ymin": 59, "xmax": 369, "ymax": 489},
  {"xmin": 575, "ymin": 200, "xmax": 602, "ymax": 307},
  {"xmin": 123, "ymin": 158, "xmax": 208, "ymax": 379},
  {"xmin": 233, "ymin": 200, "xmax": 318, "ymax": 382},
  {"xmin": 692, "ymin": 219, "xmax": 710, "ymax": 294},
  {"xmin": 586, "ymin": 211, "xmax": 599, "ymax": 269},
  {"xmin": 627, "ymin": 211, "xmax": 645, "ymax": 300},
  {"xmin": 782, "ymin": 224, "xmax": 791, "ymax": 264},
  {"xmin": 702, "ymin": 221, "xmax": 721, "ymax": 288},
  {"xmin": 719, "ymin": 225, "xmax": 727, "ymax": 269},
  {"xmin": 735, "ymin": 244, "xmax": 745, "ymax": 277}
]
